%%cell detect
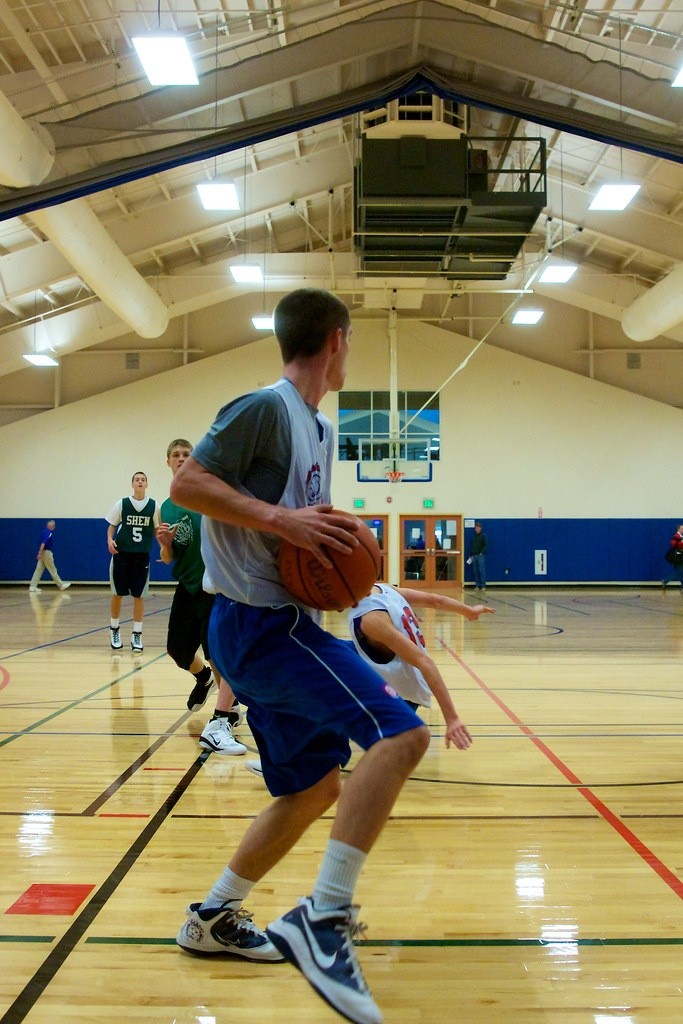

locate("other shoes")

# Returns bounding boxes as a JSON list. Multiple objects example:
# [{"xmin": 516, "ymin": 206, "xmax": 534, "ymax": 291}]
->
[
  {"xmin": 59, "ymin": 582, "xmax": 71, "ymax": 591},
  {"xmin": 29, "ymin": 591, "xmax": 41, "ymax": 598},
  {"xmin": 60, "ymin": 593, "xmax": 71, "ymax": 600},
  {"xmin": 30, "ymin": 586, "xmax": 40, "ymax": 591}
]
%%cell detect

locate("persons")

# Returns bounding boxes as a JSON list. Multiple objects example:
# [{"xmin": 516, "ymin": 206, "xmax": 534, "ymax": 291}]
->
[
  {"xmin": 470, "ymin": 523, "xmax": 487, "ymax": 591},
  {"xmin": 169, "ymin": 288, "xmax": 431, "ymax": 1024},
  {"xmin": 156, "ymin": 440, "xmax": 241, "ymax": 728},
  {"xmin": 105, "ymin": 472, "xmax": 158, "ymax": 653},
  {"xmin": 663, "ymin": 525, "xmax": 683, "ymax": 590},
  {"xmin": 197, "ymin": 676, "xmax": 246, "ymax": 756},
  {"xmin": 246, "ymin": 583, "xmax": 496, "ymax": 779},
  {"xmin": 405, "ymin": 535, "xmax": 447, "ymax": 581},
  {"xmin": 29, "ymin": 520, "xmax": 70, "ymax": 591}
]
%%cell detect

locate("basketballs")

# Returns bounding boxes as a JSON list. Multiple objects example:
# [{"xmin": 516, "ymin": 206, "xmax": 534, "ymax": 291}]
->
[{"xmin": 277, "ymin": 510, "xmax": 381, "ymax": 611}]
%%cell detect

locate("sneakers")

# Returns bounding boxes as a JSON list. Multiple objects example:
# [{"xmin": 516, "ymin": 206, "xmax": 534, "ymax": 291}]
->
[
  {"xmin": 245, "ymin": 759, "xmax": 264, "ymax": 779},
  {"xmin": 176, "ymin": 898, "xmax": 286, "ymax": 965},
  {"xmin": 110, "ymin": 626, "xmax": 123, "ymax": 650},
  {"xmin": 131, "ymin": 630, "xmax": 143, "ymax": 650},
  {"xmin": 132, "ymin": 653, "xmax": 143, "ymax": 667},
  {"xmin": 229, "ymin": 704, "xmax": 243, "ymax": 727},
  {"xmin": 187, "ymin": 667, "xmax": 218, "ymax": 713},
  {"xmin": 266, "ymin": 895, "xmax": 385, "ymax": 1024},
  {"xmin": 111, "ymin": 653, "xmax": 121, "ymax": 671},
  {"xmin": 200, "ymin": 759, "xmax": 240, "ymax": 781},
  {"xmin": 198, "ymin": 714, "xmax": 248, "ymax": 756}
]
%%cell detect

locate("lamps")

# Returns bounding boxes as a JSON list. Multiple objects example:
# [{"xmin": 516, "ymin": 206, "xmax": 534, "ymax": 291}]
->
[
  {"xmin": 22, "ymin": 288, "xmax": 61, "ymax": 366},
  {"xmin": 130, "ymin": 0, "xmax": 277, "ymax": 329},
  {"xmin": 513, "ymin": 9, "xmax": 644, "ymax": 327}
]
